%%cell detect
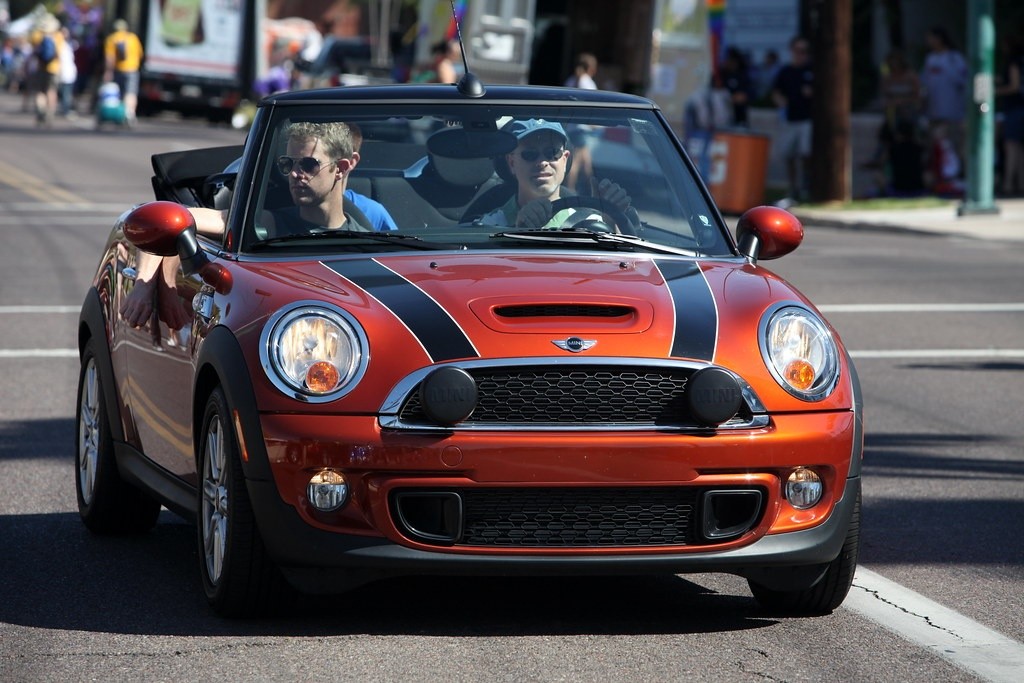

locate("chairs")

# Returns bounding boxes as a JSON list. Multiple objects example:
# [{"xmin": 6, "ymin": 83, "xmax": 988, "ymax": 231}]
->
[
  {"xmin": 414, "ymin": 127, "xmax": 502, "ymax": 222},
  {"xmin": 460, "ymin": 138, "xmax": 574, "ymax": 224},
  {"xmin": 349, "ymin": 167, "xmax": 454, "ymax": 230}
]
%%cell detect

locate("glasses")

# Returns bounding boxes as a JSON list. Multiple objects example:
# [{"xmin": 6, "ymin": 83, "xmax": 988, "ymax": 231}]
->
[
  {"xmin": 509, "ymin": 146, "xmax": 566, "ymax": 161},
  {"xmin": 277, "ymin": 156, "xmax": 337, "ymax": 177}
]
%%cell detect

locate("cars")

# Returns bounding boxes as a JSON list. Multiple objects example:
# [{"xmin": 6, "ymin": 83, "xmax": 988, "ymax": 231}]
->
[{"xmin": 76, "ymin": 86, "xmax": 865, "ymax": 612}]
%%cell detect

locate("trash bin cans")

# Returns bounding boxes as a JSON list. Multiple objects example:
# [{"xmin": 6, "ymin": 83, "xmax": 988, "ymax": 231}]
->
[{"xmin": 684, "ymin": 126, "xmax": 771, "ymax": 216}]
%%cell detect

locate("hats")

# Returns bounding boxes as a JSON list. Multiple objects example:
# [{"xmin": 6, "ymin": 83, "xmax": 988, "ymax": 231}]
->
[{"xmin": 506, "ymin": 118, "xmax": 570, "ymax": 142}]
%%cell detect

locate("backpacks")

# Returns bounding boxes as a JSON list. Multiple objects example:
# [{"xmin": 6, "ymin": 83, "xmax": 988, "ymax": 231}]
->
[
  {"xmin": 33, "ymin": 37, "xmax": 57, "ymax": 67},
  {"xmin": 114, "ymin": 43, "xmax": 126, "ymax": 62}
]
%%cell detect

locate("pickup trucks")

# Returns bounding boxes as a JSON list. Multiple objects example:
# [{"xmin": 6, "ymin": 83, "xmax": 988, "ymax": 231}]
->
[{"xmin": 287, "ymin": 36, "xmax": 410, "ymax": 89}]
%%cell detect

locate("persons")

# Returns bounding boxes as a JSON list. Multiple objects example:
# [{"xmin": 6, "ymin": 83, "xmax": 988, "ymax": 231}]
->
[
  {"xmin": 432, "ymin": 39, "xmax": 460, "ymax": 84},
  {"xmin": 565, "ymin": 53, "xmax": 599, "ymax": 191},
  {"xmin": 708, "ymin": 19, "xmax": 1024, "ymax": 209},
  {"xmin": 471, "ymin": 119, "xmax": 631, "ymax": 234},
  {"xmin": 0, "ymin": 0, "xmax": 293, "ymax": 131},
  {"xmin": 342, "ymin": 121, "xmax": 399, "ymax": 231},
  {"xmin": 120, "ymin": 123, "xmax": 374, "ymax": 328}
]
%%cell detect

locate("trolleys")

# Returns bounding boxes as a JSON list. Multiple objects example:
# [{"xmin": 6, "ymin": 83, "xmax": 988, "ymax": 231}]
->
[{"xmin": 94, "ymin": 80, "xmax": 128, "ymax": 131}]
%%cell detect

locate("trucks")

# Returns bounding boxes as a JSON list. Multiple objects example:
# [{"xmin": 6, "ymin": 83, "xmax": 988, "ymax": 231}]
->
[{"xmin": 99, "ymin": 1, "xmax": 247, "ymax": 124}]
